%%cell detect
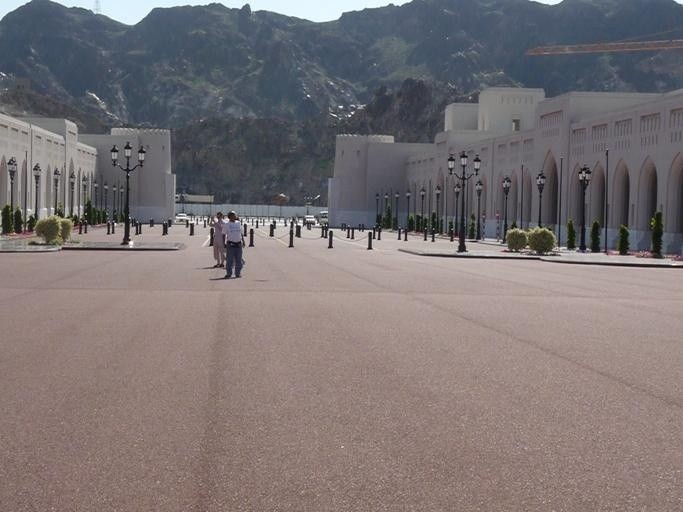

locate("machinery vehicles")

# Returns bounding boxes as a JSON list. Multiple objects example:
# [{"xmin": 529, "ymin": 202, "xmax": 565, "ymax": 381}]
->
[{"xmin": 270, "ymin": 192, "xmax": 289, "ymax": 206}]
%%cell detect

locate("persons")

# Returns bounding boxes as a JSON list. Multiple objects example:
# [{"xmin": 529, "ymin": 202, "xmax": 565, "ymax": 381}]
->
[
  {"xmin": 221, "ymin": 210, "xmax": 246, "ymax": 279},
  {"xmin": 209, "ymin": 212, "xmax": 226, "ymax": 268},
  {"xmin": 235, "ymin": 217, "xmax": 245, "ymax": 266}
]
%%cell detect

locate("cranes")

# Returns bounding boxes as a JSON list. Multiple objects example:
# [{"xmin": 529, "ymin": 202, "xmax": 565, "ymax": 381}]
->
[{"xmin": 526, "ymin": 32, "xmax": 683, "ymax": 59}]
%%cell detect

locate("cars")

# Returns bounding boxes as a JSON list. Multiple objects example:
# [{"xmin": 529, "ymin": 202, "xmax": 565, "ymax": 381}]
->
[
  {"xmin": 175, "ymin": 213, "xmax": 188, "ymax": 223},
  {"xmin": 304, "ymin": 215, "xmax": 315, "ymax": 225}
]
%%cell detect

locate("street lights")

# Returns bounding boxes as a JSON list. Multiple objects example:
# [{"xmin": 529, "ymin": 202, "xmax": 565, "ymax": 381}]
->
[
  {"xmin": 110, "ymin": 142, "xmax": 146, "ymax": 247},
  {"xmin": 372, "ymin": 164, "xmax": 596, "ymax": 252},
  {"xmin": 303, "ymin": 196, "xmax": 312, "ymax": 215},
  {"xmin": 6, "ymin": 157, "xmax": 124, "ymax": 234},
  {"xmin": 447, "ymin": 150, "xmax": 482, "ymax": 252}
]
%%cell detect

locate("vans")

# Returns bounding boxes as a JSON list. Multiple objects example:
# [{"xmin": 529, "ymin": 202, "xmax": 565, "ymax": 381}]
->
[{"xmin": 318, "ymin": 211, "xmax": 328, "ymax": 226}]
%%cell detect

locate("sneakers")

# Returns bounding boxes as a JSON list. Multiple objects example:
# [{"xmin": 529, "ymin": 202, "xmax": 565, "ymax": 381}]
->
[
  {"xmin": 235, "ymin": 275, "xmax": 241, "ymax": 278},
  {"xmin": 214, "ymin": 264, "xmax": 220, "ymax": 267},
  {"xmin": 224, "ymin": 274, "xmax": 231, "ymax": 278},
  {"xmin": 219, "ymin": 263, "xmax": 224, "ymax": 267}
]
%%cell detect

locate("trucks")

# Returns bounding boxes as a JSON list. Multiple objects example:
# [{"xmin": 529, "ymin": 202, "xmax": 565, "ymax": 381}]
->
[{"xmin": 174, "ymin": 193, "xmax": 215, "ymax": 204}]
